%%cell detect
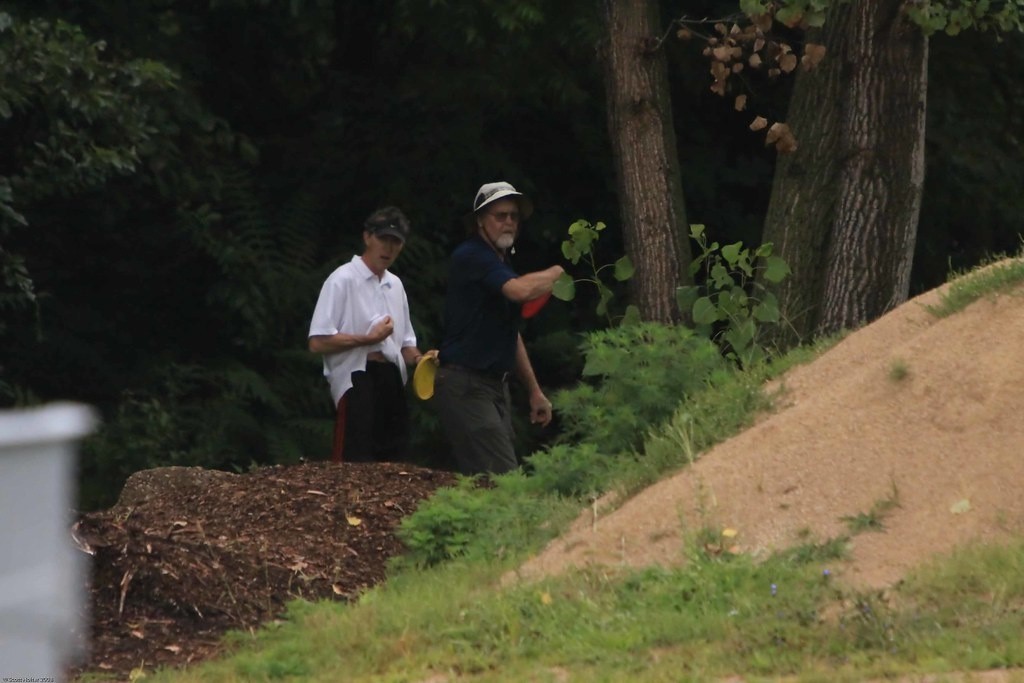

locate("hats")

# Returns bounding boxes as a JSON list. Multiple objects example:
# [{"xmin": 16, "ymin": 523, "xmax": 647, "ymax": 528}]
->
[
  {"xmin": 473, "ymin": 181, "xmax": 523, "ymax": 213},
  {"xmin": 373, "ymin": 217, "xmax": 409, "ymax": 245}
]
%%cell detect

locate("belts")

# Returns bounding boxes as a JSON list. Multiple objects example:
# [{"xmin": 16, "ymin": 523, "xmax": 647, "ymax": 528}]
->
[{"xmin": 441, "ymin": 363, "xmax": 511, "ymax": 384}]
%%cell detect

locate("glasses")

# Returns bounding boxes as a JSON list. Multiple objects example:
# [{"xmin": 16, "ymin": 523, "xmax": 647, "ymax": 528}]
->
[{"xmin": 487, "ymin": 211, "xmax": 521, "ymax": 221}]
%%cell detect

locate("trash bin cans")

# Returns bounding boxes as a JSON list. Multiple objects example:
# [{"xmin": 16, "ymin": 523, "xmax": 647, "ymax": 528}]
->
[{"xmin": 0, "ymin": 398, "xmax": 96, "ymax": 682}]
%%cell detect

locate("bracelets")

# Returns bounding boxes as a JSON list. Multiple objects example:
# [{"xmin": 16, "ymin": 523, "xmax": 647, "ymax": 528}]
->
[{"xmin": 414, "ymin": 355, "xmax": 421, "ymax": 364}]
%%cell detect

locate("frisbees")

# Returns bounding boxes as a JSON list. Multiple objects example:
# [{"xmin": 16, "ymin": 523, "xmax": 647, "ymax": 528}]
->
[
  {"xmin": 413, "ymin": 352, "xmax": 436, "ymax": 401},
  {"xmin": 522, "ymin": 289, "xmax": 554, "ymax": 318}
]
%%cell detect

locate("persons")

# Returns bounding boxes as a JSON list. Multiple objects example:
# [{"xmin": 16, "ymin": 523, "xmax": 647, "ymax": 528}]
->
[
  {"xmin": 435, "ymin": 182, "xmax": 564, "ymax": 474},
  {"xmin": 308, "ymin": 207, "xmax": 440, "ymax": 463}
]
